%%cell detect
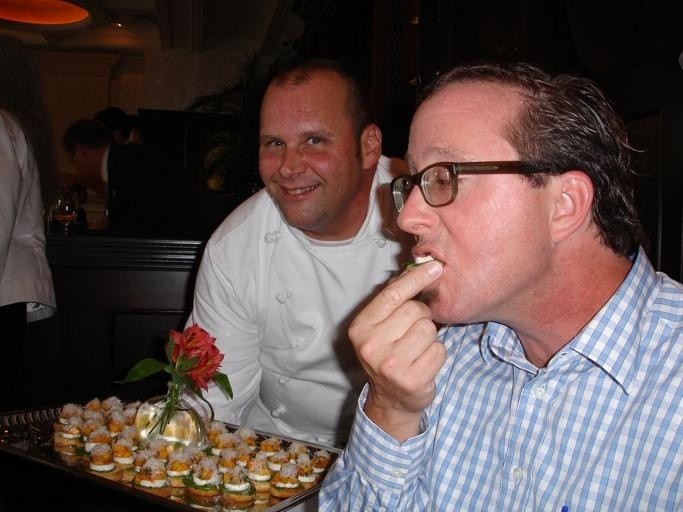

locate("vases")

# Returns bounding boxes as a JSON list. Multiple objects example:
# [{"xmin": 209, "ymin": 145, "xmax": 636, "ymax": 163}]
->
[{"xmin": 133, "ymin": 382, "xmax": 204, "ymax": 451}]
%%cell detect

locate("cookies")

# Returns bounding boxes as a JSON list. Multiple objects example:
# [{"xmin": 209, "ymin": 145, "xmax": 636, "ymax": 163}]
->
[
  {"xmin": 405, "ymin": 259, "xmax": 444, "ymax": 272},
  {"xmin": 52, "ymin": 396, "xmax": 330, "ymax": 503}
]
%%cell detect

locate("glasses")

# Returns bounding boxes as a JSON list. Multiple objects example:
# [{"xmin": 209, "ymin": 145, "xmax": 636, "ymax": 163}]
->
[{"xmin": 389, "ymin": 160, "xmax": 555, "ymax": 214}]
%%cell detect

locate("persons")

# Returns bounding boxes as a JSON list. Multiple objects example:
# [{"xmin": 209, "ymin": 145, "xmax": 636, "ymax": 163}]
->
[
  {"xmin": 62, "ymin": 107, "xmax": 247, "ymax": 238},
  {"xmin": 317, "ymin": 62, "xmax": 681, "ymax": 510},
  {"xmin": 168, "ymin": 58, "xmax": 408, "ymax": 449},
  {"xmin": 1, "ymin": 34, "xmax": 59, "ymax": 512}
]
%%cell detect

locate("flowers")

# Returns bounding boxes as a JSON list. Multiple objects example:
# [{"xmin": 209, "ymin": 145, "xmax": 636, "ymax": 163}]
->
[{"xmin": 115, "ymin": 324, "xmax": 233, "ymax": 423}]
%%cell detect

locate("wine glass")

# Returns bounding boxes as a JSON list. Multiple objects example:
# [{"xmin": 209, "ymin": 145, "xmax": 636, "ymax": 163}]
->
[{"xmin": 54, "ymin": 189, "xmax": 79, "ymax": 236}]
